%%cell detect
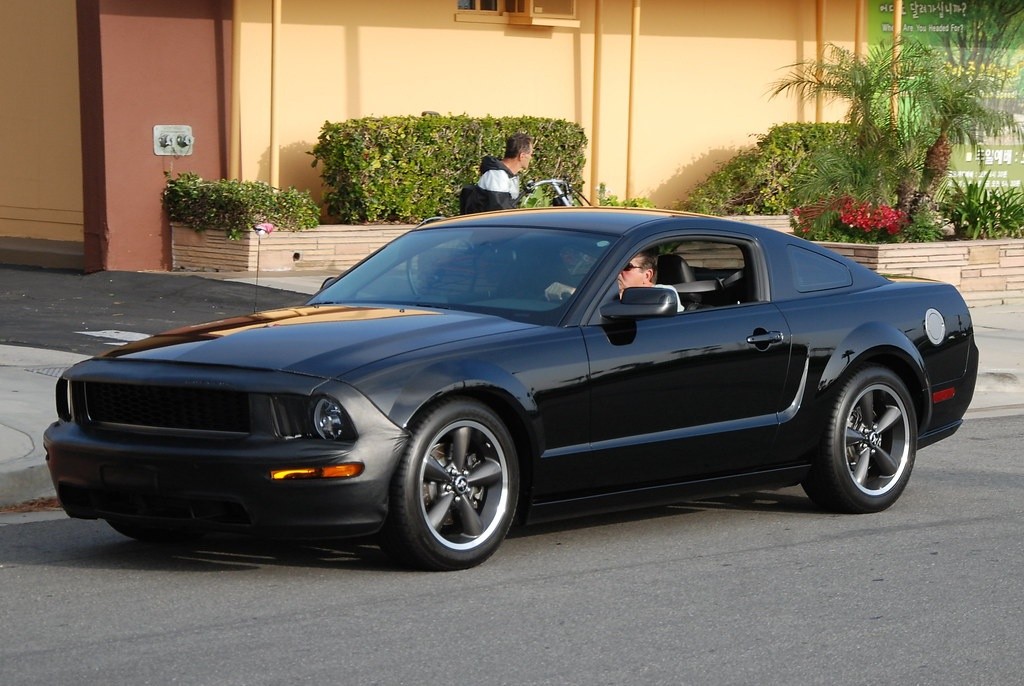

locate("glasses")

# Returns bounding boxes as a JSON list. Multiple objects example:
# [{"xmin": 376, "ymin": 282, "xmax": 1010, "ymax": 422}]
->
[{"xmin": 623, "ymin": 263, "xmax": 642, "ymax": 271}]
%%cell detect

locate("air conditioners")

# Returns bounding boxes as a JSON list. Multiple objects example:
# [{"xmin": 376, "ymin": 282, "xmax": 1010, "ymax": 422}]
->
[{"xmin": 506, "ymin": 0, "xmax": 577, "ymax": 20}]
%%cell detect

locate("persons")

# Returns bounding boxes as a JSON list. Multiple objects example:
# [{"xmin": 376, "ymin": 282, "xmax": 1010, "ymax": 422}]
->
[
  {"xmin": 458, "ymin": 133, "xmax": 534, "ymax": 216},
  {"xmin": 545, "ymin": 246, "xmax": 685, "ymax": 314}
]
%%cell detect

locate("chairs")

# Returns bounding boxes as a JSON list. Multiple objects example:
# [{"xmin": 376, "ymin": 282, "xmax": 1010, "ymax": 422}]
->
[{"xmin": 657, "ymin": 253, "xmax": 714, "ymax": 311}]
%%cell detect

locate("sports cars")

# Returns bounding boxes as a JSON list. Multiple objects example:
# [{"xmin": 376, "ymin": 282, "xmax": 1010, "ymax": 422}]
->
[{"xmin": 41, "ymin": 205, "xmax": 980, "ymax": 574}]
